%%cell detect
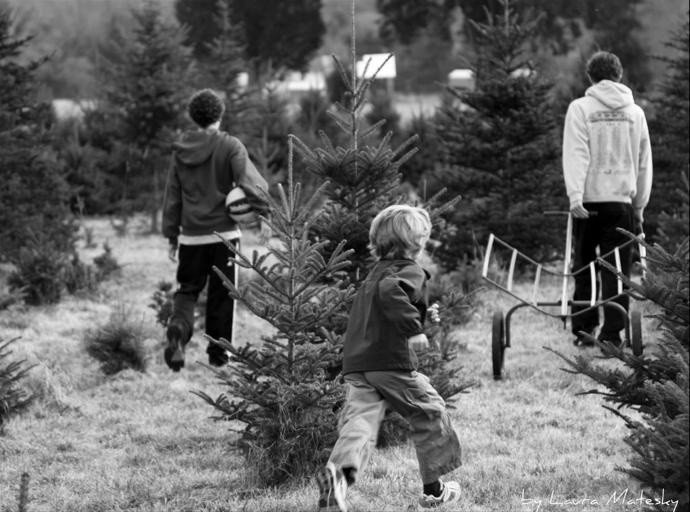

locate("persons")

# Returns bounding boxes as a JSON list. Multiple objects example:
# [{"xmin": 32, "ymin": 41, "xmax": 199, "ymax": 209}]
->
[
  {"xmin": 161, "ymin": 89, "xmax": 271, "ymax": 372},
  {"xmin": 562, "ymin": 52, "xmax": 654, "ymax": 359},
  {"xmin": 317, "ymin": 205, "xmax": 463, "ymax": 512}
]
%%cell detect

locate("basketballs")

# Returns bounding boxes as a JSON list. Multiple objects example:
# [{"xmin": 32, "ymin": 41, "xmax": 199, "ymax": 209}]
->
[{"xmin": 224, "ymin": 187, "xmax": 258, "ymax": 225}]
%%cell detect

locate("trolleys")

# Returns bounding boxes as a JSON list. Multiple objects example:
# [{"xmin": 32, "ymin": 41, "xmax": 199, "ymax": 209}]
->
[{"xmin": 477, "ymin": 206, "xmax": 653, "ymax": 377}]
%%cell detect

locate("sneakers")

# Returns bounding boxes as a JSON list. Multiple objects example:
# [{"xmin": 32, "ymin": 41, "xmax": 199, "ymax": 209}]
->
[
  {"xmin": 208, "ymin": 352, "xmax": 228, "ymax": 366},
  {"xmin": 417, "ymin": 481, "xmax": 462, "ymax": 511},
  {"xmin": 573, "ymin": 334, "xmax": 595, "ymax": 347},
  {"xmin": 314, "ymin": 462, "xmax": 350, "ymax": 512},
  {"xmin": 164, "ymin": 321, "xmax": 184, "ymax": 371}
]
